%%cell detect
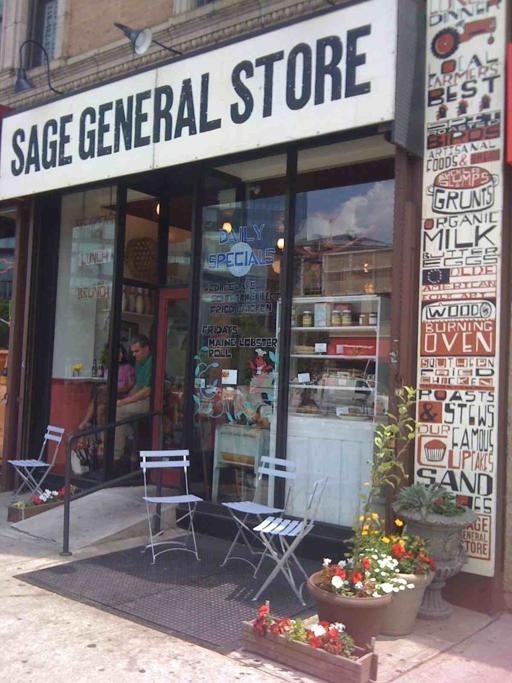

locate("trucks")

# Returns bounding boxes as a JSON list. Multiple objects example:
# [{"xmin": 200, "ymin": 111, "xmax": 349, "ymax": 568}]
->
[{"xmin": 316, "ymin": 371, "xmax": 390, "ymax": 415}]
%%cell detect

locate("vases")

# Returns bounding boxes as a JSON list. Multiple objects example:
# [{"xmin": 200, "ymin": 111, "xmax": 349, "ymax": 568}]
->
[
  {"xmin": 307, "ymin": 568, "xmax": 393, "ymax": 644},
  {"xmin": 8, "ymin": 502, "xmax": 64, "ymax": 523},
  {"xmin": 240, "ymin": 613, "xmax": 378, "ymax": 683},
  {"xmin": 376, "ymin": 571, "xmax": 435, "ymax": 636}
]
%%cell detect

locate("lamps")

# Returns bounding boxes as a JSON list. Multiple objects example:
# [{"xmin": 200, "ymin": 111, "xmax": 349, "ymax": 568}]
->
[
  {"xmin": 114, "ymin": 21, "xmax": 184, "ymax": 56},
  {"xmin": 15, "ymin": 39, "xmax": 63, "ymax": 94}
]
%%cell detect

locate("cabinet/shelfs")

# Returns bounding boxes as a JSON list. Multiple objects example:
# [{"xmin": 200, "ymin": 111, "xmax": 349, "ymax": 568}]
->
[
  {"xmin": 321, "ymin": 247, "xmax": 395, "ymax": 297},
  {"xmin": 267, "ymin": 294, "xmax": 392, "ymax": 532}
]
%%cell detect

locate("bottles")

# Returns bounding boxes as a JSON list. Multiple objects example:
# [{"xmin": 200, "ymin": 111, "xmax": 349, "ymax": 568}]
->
[
  {"xmin": 110, "ymin": 283, "xmax": 158, "ymax": 315},
  {"xmin": 289, "ymin": 305, "xmax": 377, "ymax": 328}
]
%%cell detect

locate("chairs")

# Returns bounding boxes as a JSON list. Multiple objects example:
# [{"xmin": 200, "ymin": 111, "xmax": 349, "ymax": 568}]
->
[
  {"xmin": 221, "ymin": 455, "xmax": 296, "ymax": 579},
  {"xmin": 139, "ymin": 449, "xmax": 204, "ymax": 565},
  {"xmin": 8, "ymin": 425, "xmax": 64, "ymax": 501},
  {"xmin": 250, "ymin": 476, "xmax": 329, "ymax": 606}
]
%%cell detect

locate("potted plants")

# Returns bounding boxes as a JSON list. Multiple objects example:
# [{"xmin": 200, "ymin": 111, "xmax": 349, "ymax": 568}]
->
[{"xmin": 390, "ymin": 482, "xmax": 478, "ymax": 620}]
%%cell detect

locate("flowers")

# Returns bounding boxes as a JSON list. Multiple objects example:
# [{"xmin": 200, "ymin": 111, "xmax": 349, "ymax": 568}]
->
[
  {"xmin": 254, "ymin": 605, "xmax": 354, "ymax": 658},
  {"xmin": 11, "ymin": 487, "xmax": 65, "ymax": 509},
  {"xmin": 321, "ymin": 547, "xmax": 416, "ymax": 599},
  {"xmin": 359, "ymin": 512, "xmax": 437, "ymax": 576}
]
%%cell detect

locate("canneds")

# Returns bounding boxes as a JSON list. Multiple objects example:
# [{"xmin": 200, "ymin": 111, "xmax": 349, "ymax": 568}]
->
[{"xmin": 302, "ymin": 310, "xmax": 377, "ymax": 327}]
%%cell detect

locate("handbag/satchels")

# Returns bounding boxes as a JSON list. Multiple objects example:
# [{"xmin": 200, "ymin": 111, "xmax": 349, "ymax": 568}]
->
[{"xmin": 70, "ymin": 446, "xmax": 91, "ymax": 474}]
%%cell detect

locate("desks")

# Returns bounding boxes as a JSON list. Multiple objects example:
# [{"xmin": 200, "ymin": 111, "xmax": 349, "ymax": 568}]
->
[{"xmin": 212, "ymin": 423, "xmax": 270, "ymax": 505}]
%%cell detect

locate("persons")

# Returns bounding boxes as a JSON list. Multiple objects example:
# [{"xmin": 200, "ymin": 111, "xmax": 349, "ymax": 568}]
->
[
  {"xmin": 97, "ymin": 332, "xmax": 157, "ymax": 474},
  {"xmin": 75, "ymin": 342, "xmax": 134, "ymax": 456}
]
